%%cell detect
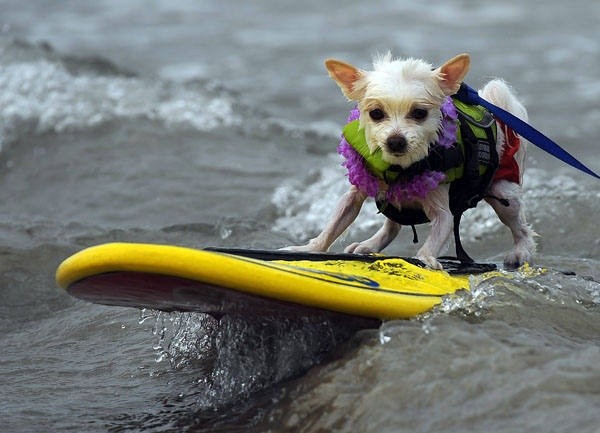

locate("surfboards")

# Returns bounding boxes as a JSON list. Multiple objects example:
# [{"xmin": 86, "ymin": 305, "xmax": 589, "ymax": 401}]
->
[{"xmin": 57, "ymin": 241, "xmax": 578, "ymax": 326}]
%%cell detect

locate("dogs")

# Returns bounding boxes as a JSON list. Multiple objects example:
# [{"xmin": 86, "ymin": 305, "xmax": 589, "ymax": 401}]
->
[{"xmin": 278, "ymin": 51, "xmax": 542, "ymax": 270}]
